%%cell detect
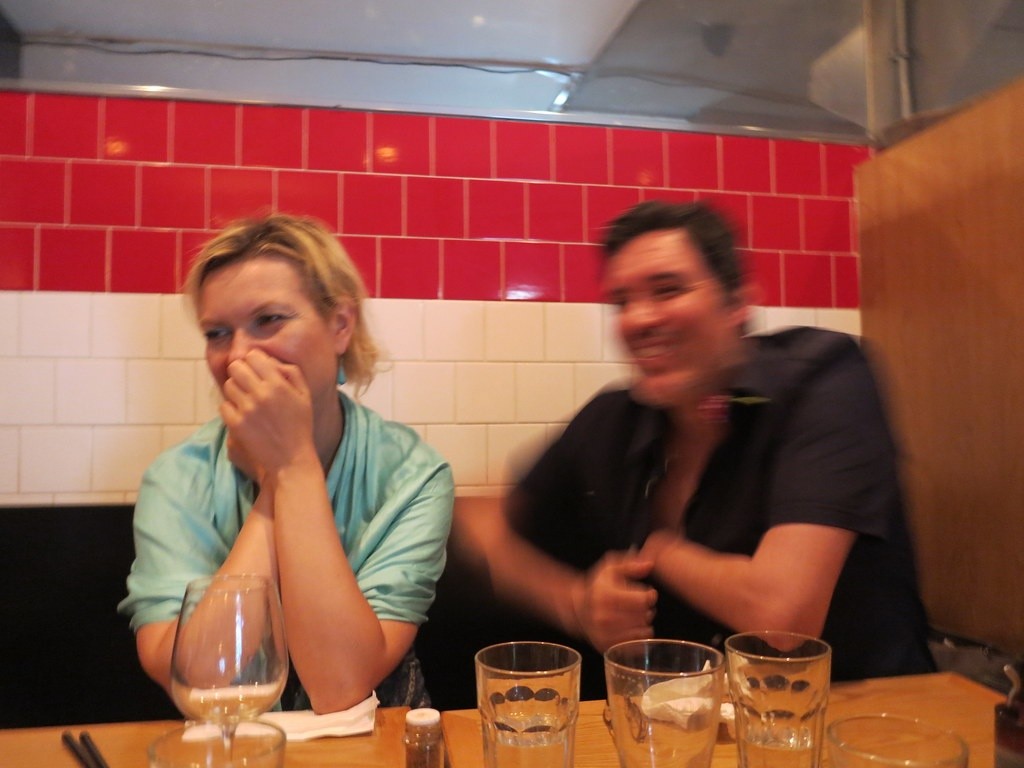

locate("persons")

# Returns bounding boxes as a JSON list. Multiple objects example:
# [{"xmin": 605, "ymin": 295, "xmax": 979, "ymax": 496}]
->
[
  {"xmin": 453, "ymin": 194, "xmax": 938, "ymax": 680},
  {"xmin": 114, "ymin": 211, "xmax": 455, "ymax": 719}
]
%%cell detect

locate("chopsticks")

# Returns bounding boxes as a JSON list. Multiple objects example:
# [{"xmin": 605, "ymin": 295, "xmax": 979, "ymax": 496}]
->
[{"xmin": 62, "ymin": 730, "xmax": 110, "ymax": 768}]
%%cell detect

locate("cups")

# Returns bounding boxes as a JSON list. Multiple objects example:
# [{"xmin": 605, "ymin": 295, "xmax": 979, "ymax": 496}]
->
[
  {"xmin": 603, "ymin": 639, "xmax": 725, "ymax": 768},
  {"xmin": 474, "ymin": 641, "xmax": 581, "ymax": 768},
  {"xmin": 146, "ymin": 719, "xmax": 286, "ymax": 768},
  {"xmin": 826, "ymin": 713, "xmax": 968, "ymax": 768},
  {"xmin": 994, "ymin": 703, "xmax": 1024, "ymax": 768},
  {"xmin": 724, "ymin": 630, "xmax": 833, "ymax": 768}
]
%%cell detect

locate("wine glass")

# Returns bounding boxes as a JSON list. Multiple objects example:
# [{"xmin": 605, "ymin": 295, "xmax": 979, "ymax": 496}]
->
[{"xmin": 169, "ymin": 574, "xmax": 289, "ymax": 768}]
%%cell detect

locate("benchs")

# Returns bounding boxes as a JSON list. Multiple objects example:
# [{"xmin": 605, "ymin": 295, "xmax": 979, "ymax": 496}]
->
[{"xmin": 0, "ymin": 504, "xmax": 608, "ymax": 728}]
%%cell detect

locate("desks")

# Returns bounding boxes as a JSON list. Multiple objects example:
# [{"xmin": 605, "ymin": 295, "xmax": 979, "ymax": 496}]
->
[{"xmin": 0, "ymin": 671, "xmax": 1008, "ymax": 768}]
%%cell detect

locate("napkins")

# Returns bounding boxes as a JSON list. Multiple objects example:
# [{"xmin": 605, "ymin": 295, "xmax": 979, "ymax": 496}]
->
[
  {"xmin": 641, "ymin": 660, "xmax": 751, "ymax": 739},
  {"xmin": 181, "ymin": 689, "xmax": 380, "ymax": 742}
]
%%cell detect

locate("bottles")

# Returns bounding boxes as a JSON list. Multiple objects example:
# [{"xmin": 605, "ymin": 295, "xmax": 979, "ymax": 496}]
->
[{"xmin": 404, "ymin": 707, "xmax": 450, "ymax": 768}]
